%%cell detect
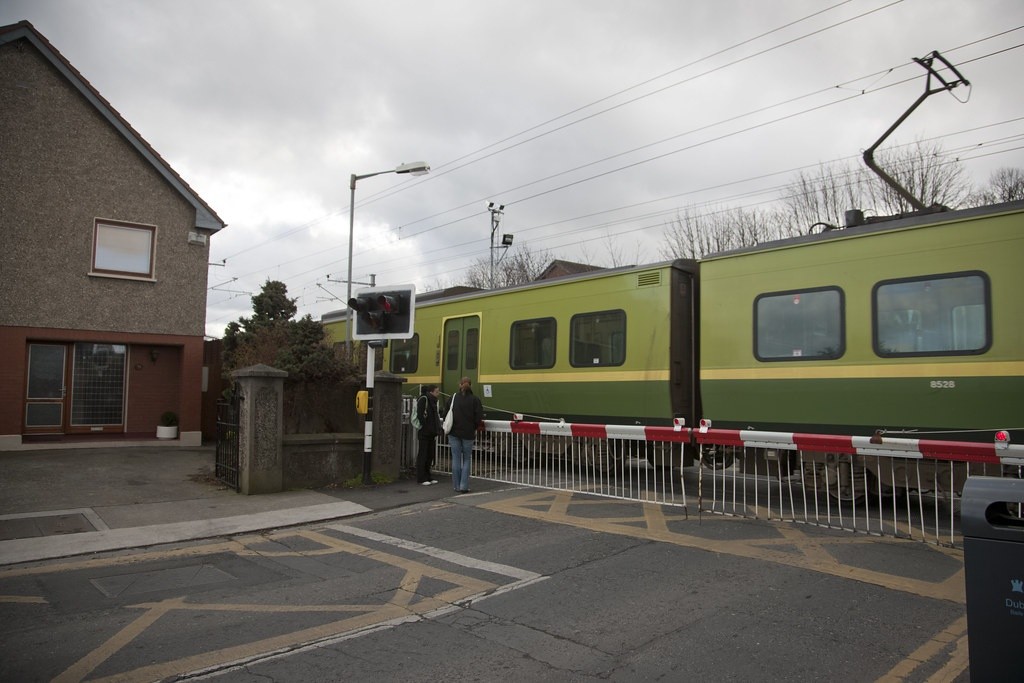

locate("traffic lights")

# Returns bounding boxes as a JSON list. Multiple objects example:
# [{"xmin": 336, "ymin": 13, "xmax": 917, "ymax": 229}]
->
[{"xmin": 347, "ymin": 284, "xmax": 415, "ymax": 341}]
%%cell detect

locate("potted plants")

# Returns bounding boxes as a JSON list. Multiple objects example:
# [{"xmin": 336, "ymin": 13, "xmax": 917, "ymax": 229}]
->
[{"xmin": 156, "ymin": 412, "xmax": 178, "ymax": 441}]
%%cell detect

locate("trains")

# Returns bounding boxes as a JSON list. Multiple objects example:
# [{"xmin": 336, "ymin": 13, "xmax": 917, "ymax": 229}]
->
[{"xmin": 314, "ymin": 50, "xmax": 1024, "ymax": 515}]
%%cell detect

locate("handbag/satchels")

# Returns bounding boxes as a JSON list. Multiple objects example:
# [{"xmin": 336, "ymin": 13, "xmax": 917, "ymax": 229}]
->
[
  {"xmin": 410, "ymin": 395, "xmax": 428, "ymax": 430},
  {"xmin": 442, "ymin": 392, "xmax": 457, "ymax": 434}
]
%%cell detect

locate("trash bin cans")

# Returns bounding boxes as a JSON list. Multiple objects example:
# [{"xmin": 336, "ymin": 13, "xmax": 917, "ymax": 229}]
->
[{"xmin": 960, "ymin": 475, "xmax": 1024, "ymax": 683}]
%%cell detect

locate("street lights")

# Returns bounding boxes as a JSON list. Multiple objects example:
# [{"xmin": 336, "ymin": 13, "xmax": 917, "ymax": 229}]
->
[
  {"xmin": 488, "ymin": 201, "xmax": 514, "ymax": 288},
  {"xmin": 342, "ymin": 161, "xmax": 432, "ymax": 365}
]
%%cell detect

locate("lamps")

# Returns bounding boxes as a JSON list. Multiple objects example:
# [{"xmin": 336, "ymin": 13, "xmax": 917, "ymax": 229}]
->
[{"xmin": 151, "ymin": 349, "xmax": 161, "ymax": 363}]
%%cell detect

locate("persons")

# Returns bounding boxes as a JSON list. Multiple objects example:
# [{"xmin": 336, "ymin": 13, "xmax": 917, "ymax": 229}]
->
[
  {"xmin": 416, "ymin": 385, "xmax": 442, "ymax": 486},
  {"xmin": 443, "ymin": 377, "xmax": 483, "ymax": 492}
]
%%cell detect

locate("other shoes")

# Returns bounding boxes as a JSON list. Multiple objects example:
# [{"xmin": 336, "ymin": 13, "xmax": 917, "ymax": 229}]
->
[
  {"xmin": 431, "ymin": 479, "xmax": 438, "ymax": 483},
  {"xmin": 454, "ymin": 487, "xmax": 460, "ymax": 494},
  {"xmin": 460, "ymin": 488, "xmax": 471, "ymax": 494},
  {"xmin": 419, "ymin": 481, "xmax": 431, "ymax": 485}
]
